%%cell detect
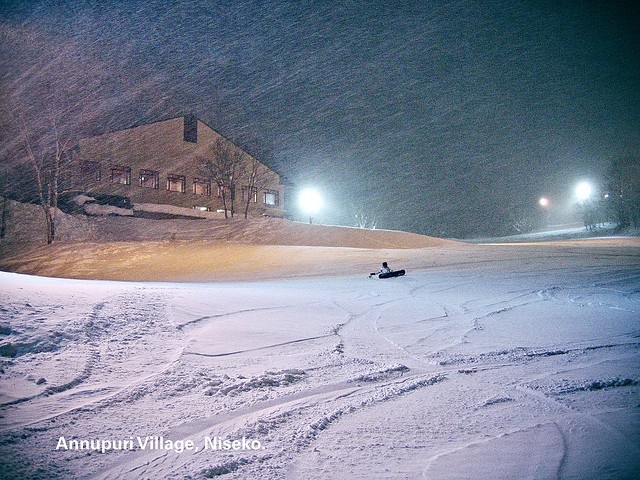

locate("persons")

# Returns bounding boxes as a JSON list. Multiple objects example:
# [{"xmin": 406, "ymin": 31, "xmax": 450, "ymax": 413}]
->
[{"xmin": 370, "ymin": 262, "xmax": 391, "ymax": 276}]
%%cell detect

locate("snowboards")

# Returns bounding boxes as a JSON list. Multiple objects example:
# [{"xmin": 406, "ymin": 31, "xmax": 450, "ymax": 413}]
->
[{"xmin": 379, "ymin": 270, "xmax": 405, "ymax": 279}]
